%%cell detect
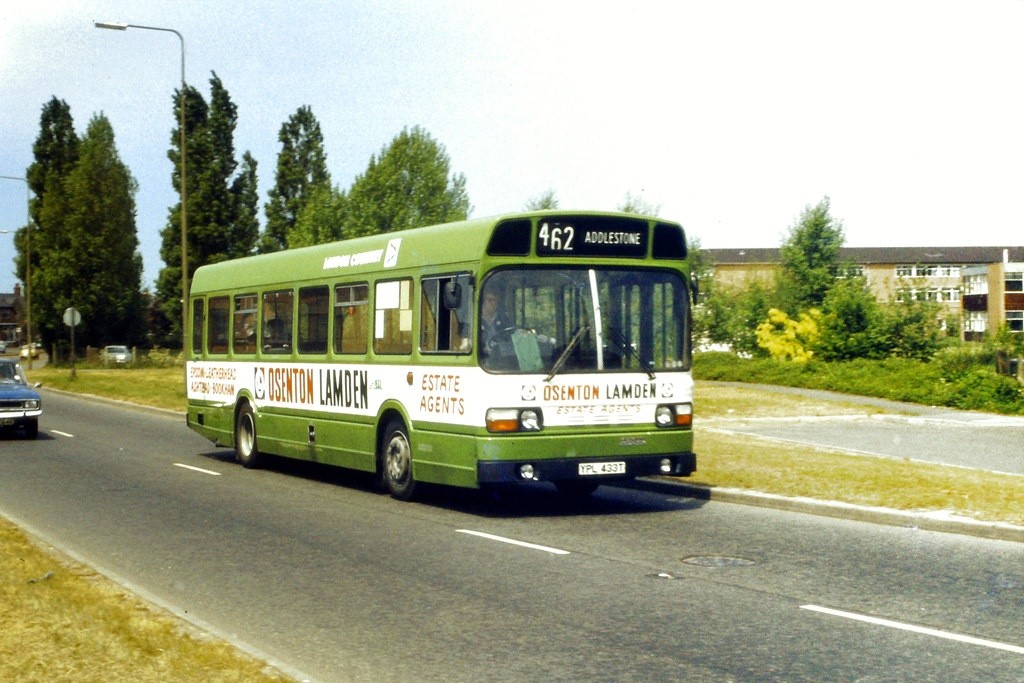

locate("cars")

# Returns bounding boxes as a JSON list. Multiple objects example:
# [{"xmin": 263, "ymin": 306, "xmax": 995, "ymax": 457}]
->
[
  {"xmin": 20, "ymin": 345, "xmax": 40, "ymax": 359},
  {"xmin": 100, "ymin": 345, "xmax": 131, "ymax": 364},
  {"xmin": 0, "ymin": 358, "xmax": 43, "ymax": 434},
  {"xmin": 0, "ymin": 341, "xmax": 6, "ymax": 352}
]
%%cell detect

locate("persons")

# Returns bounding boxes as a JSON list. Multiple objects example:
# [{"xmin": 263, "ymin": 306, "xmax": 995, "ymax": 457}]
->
[
  {"xmin": 266, "ymin": 319, "xmax": 291, "ymax": 348},
  {"xmin": 461, "ymin": 290, "xmax": 513, "ymax": 353}
]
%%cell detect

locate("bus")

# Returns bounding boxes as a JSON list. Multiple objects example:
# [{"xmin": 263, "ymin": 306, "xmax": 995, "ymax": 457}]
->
[{"xmin": 186, "ymin": 211, "xmax": 699, "ymax": 502}]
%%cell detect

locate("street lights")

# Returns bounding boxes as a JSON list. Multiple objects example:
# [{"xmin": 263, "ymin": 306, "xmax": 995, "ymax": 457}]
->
[{"xmin": 93, "ymin": 19, "xmax": 188, "ymax": 341}]
